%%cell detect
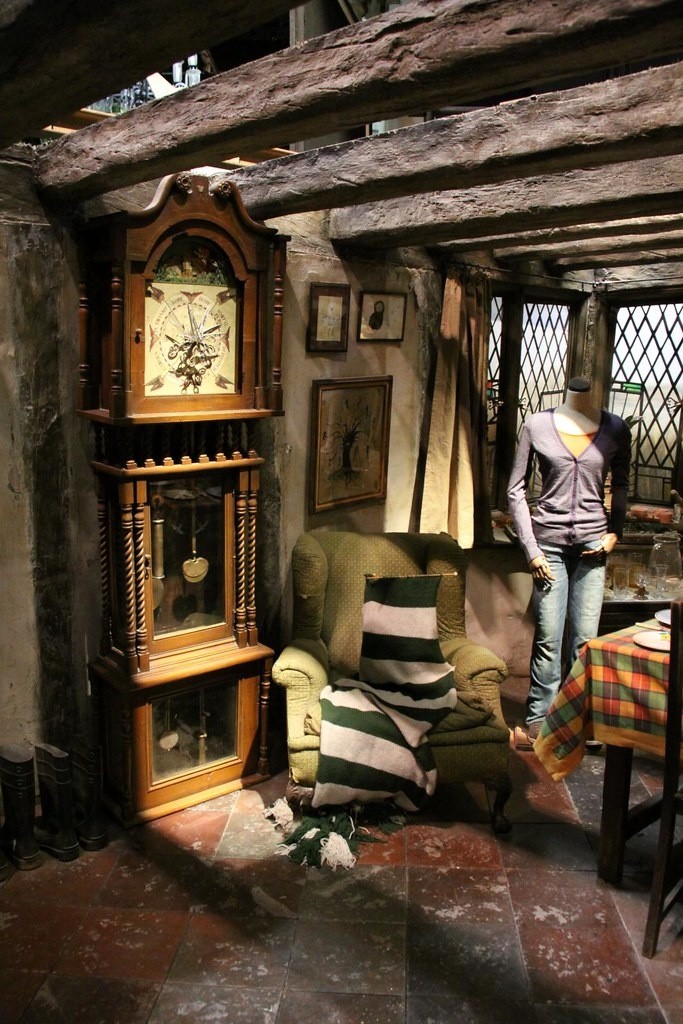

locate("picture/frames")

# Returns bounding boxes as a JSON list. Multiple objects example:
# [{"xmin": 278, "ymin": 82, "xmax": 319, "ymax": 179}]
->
[
  {"xmin": 308, "ymin": 375, "xmax": 394, "ymax": 516},
  {"xmin": 306, "ymin": 282, "xmax": 351, "ymax": 354},
  {"xmin": 356, "ymin": 290, "xmax": 408, "ymax": 342}
]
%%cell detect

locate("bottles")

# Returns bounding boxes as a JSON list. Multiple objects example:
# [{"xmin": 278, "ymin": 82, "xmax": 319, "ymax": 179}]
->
[{"xmin": 649, "ymin": 535, "xmax": 682, "ymax": 590}]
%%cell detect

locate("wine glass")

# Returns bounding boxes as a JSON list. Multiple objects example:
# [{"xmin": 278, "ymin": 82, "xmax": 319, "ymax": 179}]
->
[
  {"xmin": 651, "ymin": 564, "xmax": 669, "ymax": 599},
  {"xmin": 634, "ymin": 572, "xmax": 649, "ymax": 595},
  {"xmin": 605, "ymin": 576, "xmax": 612, "ymax": 593},
  {"xmin": 636, "ymin": 577, "xmax": 647, "ymax": 600}
]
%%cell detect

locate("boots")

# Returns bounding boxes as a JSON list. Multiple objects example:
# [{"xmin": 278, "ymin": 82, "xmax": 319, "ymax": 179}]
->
[
  {"xmin": 32, "ymin": 733, "xmax": 107, "ymax": 862},
  {"xmin": 0, "ymin": 742, "xmax": 42, "ymax": 881}
]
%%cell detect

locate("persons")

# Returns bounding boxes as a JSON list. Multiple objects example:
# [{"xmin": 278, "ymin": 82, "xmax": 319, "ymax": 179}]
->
[{"xmin": 506, "ymin": 377, "xmax": 633, "ymax": 745}]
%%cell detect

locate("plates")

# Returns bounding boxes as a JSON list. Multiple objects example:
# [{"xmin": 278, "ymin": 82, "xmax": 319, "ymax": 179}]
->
[
  {"xmin": 655, "ymin": 609, "xmax": 671, "ymax": 625},
  {"xmin": 632, "ymin": 632, "xmax": 670, "ymax": 651}
]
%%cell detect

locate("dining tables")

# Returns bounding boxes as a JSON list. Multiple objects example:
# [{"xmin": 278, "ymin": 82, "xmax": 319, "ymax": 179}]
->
[{"xmin": 587, "ymin": 617, "xmax": 683, "ymax": 890}]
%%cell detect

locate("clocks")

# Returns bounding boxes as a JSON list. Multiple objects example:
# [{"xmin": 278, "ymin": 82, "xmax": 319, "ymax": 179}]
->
[{"xmin": 72, "ymin": 172, "xmax": 291, "ymax": 422}]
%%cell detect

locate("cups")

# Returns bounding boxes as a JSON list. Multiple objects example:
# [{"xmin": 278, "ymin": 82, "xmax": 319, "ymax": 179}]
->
[
  {"xmin": 628, "ymin": 564, "xmax": 645, "ymax": 589},
  {"xmin": 608, "ymin": 553, "xmax": 623, "ymax": 575},
  {"xmin": 614, "ymin": 567, "xmax": 629, "ymax": 599},
  {"xmin": 628, "ymin": 553, "xmax": 643, "ymax": 563}
]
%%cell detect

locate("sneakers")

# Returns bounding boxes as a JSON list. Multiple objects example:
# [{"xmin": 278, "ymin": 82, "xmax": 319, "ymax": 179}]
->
[
  {"xmin": 585, "ymin": 735, "xmax": 603, "ymax": 745},
  {"xmin": 527, "ymin": 721, "xmax": 544, "ymax": 743}
]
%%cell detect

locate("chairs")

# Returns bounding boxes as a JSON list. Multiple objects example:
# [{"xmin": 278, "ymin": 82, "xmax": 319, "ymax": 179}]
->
[{"xmin": 641, "ymin": 596, "xmax": 683, "ymax": 959}]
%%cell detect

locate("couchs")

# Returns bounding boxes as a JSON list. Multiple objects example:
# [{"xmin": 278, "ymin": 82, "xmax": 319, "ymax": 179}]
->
[{"xmin": 271, "ymin": 530, "xmax": 515, "ymax": 833}]
